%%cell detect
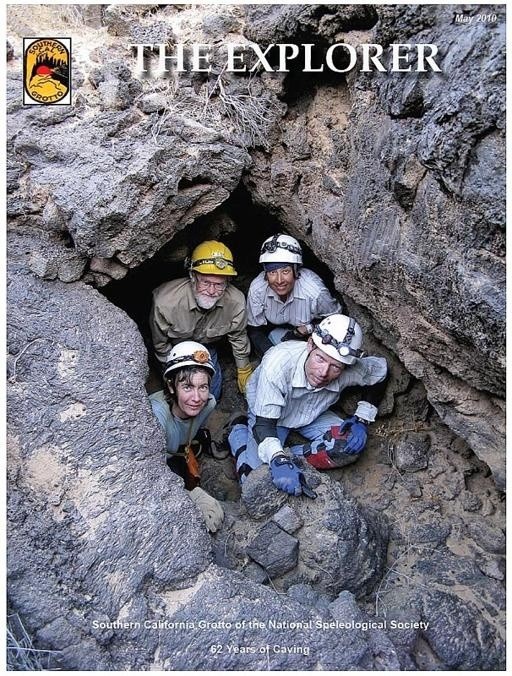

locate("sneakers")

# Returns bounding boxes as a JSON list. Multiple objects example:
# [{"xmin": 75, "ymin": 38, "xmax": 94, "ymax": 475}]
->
[{"xmin": 210, "ymin": 409, "xmax": 247, "ymax": 460}]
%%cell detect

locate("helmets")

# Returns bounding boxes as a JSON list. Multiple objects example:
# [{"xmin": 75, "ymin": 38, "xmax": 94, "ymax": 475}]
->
[
  {"xmin": 190, "ymin": 240, "xmax": 238, "ymax": 277},
  {"xmin": 312, "ymin": 314, "xmax": 364, "ymax": 366},
  {"xmin": 163, "ymin": 340, "xmax": 216, "ymax": 383},
  {"xmin": 258, "ymin": 234, "xmax": 304, "ymax": 265}
]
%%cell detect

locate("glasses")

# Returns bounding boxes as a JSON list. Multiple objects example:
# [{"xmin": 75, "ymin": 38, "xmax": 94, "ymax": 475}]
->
[{"xmin": 193, "ymin": 271, "xmax": 227, "ymax": 291}]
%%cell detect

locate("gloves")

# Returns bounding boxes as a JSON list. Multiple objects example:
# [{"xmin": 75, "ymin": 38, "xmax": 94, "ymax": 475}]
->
[
  {"xmin": 340, "ymin": 415, "xmax": 368, "ymax": 456},
  {"xmin": 270, "ymin": 455, "xmax": 308, "ymax": 496},
  {"xmin": 190, "ymin": 487, "xmax": 224, "ymax": 533},
  {"xmin": 236, "ymin": 367, "xmax": 254, "ymax": 393},
  {"xmin": 281, "ymin": 331, "xmax": 302, "ymax": 343}
]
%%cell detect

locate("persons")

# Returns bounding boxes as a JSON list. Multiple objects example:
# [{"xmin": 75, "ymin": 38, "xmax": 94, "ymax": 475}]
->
[
  {"xmin": 244, "ymin": 231, "xmax": 345, "ymax": 369},
  {"xmin": 144, "ymin": 238, "xmax": 255, "ymax": 404},
  {"xmin": 146, "ymin": 340, "xmax": 226, "ymax": 537},
  {"xmin": 211, "ymin": 313, "xmax": 390, "ymax": 500}
]
